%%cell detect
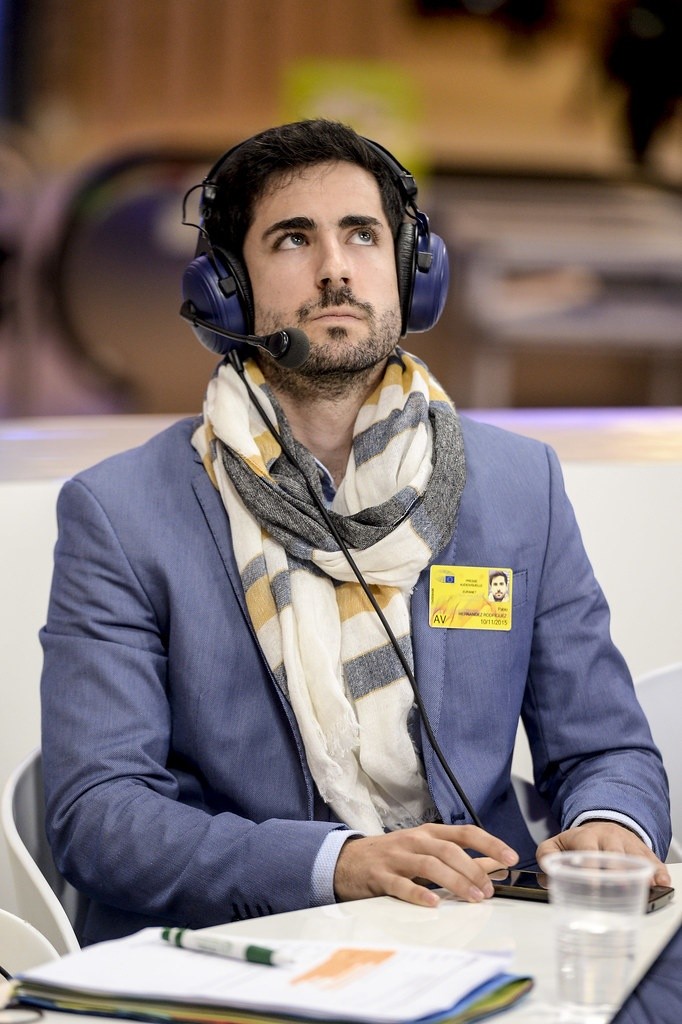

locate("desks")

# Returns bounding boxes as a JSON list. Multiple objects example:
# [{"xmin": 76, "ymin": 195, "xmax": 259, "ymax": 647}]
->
[{"xmin": 0, "ymin": 859, "xmax": 682, "ymax": 1024}]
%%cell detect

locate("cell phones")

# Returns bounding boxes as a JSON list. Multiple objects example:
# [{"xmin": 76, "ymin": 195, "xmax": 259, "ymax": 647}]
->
[{"xmin": 481, "ymin": 869, "xmax": 676, "ymax": 912}]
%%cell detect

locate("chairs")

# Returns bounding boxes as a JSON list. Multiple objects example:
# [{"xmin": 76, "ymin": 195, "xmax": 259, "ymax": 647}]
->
[
  {"xmin": 0, "ymin": 902, "xmax": 59, "ymax": 982},
  {"xmin": 4, "ymin": 740, "xmax": 89, "ymax": 952}
]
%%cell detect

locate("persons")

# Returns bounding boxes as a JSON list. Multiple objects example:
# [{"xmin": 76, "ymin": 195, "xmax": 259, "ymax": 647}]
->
[
  {"xmin": 39, "ymin": 117, "xmax": 681, "ymax": 1017},
  {"xmin": 487, "ymin": 571, "xmax": 511, "ymax": 604}
]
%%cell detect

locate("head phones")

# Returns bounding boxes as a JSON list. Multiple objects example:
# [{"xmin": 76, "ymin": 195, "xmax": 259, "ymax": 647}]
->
[{"xmin": 182, "ymin": 128, "xmax": 451, "ymax": 357}]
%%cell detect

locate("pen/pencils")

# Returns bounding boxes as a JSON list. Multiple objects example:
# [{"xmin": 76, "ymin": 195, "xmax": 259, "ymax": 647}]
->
[{"xmin": 162, "ymin": 926, "xmax": 295, "ymax": 967}]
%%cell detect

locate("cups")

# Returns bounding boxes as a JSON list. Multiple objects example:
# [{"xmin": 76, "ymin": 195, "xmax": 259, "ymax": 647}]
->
[{"xmin": 535, "ymin": 847, "xmax": 655, "ymax": 1010}]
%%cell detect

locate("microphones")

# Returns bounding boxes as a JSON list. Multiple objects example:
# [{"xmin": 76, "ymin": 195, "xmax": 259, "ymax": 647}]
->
[{"xmin": 178, "ymin": 301, "xmax": 310, "ymax": 368}]
